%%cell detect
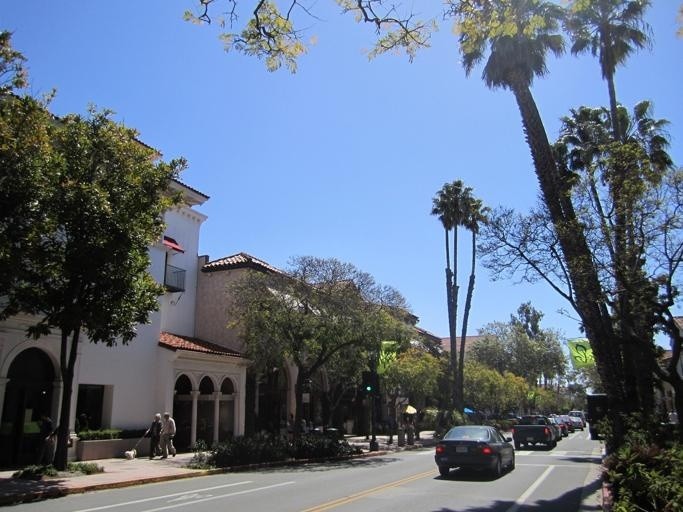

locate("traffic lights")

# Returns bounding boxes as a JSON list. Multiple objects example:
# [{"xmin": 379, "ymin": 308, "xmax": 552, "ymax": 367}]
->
[{"xmin": 361, "ymin": 373, "xmax": 374, "ymax": 396}]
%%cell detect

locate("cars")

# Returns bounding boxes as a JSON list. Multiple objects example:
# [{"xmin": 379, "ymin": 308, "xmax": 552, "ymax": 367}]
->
[
  {"xmin": 432, "ymin": 423, "xmax": 516, "ymax": 478},
  {"xmin": 543, "ymin": 410, "xmax": 586, "ymax": 439}
]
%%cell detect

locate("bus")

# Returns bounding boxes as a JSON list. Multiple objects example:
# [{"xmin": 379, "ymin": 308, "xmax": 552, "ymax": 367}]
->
[{"xmin": 585, "ymin": 391, "xmax": 608, "ymax": 439}]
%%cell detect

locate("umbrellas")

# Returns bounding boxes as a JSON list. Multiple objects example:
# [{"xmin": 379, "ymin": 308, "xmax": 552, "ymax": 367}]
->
[{"xmin": 402, "ymin": 405, "xmax": 417, "ymax": 414}]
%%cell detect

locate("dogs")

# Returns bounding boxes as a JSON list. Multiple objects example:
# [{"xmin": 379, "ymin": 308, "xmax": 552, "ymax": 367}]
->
[{"xmin": 125, "ymin": 448, "xmax": 137, "ymax": 460}]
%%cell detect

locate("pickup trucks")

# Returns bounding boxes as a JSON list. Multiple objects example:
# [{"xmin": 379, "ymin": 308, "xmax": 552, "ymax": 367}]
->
[{"xmin": 509, "ymin": 414, "xmax": 558, "ymax": 451}]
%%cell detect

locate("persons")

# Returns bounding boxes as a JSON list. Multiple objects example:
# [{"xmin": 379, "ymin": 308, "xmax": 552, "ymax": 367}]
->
[
  {"xmin": 79, "ymin": 413, "xmax": 88, "ymax": 432},
  {"xmin": 37, "ymin": 408, "xmax": 57, "ymax": 465},
  {"xmin": 149, "ymin": 413, "xmax": 163, "ymax": 460},
  {"xmin": 404, "ymin": 409, "xmax": 424, "ymax": 440},
  {"xmin": 48, "ymin": 426, "xmax": 73, "ymax": 447},
  {"xmin": 280, "ymin": 413, "xmax": 314, "ymax": 442},
  {"xmin": 161, "ymin": 412, "xmax": 177, "ymax": 459}
]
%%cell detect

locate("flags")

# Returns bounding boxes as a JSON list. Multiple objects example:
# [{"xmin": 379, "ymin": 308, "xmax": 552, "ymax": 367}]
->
[{"xmin": 567, "ymin": 339, "xmax": 595, "ymax": 370}]
